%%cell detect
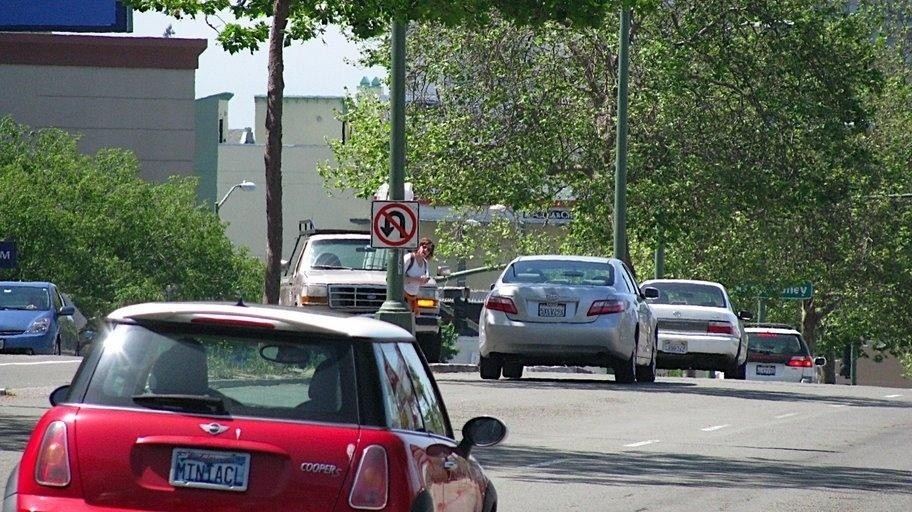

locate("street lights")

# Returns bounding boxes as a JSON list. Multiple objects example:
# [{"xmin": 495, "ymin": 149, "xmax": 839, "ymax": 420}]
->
[{"xmin": 213, "ymin": 178, "xmax": 256, "ymax": 221}]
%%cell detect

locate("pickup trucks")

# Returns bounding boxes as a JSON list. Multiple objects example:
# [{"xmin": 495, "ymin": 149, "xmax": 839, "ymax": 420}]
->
[{"xmin": 263, "ymin": 219, "xmax": 443, "ymax": 363}]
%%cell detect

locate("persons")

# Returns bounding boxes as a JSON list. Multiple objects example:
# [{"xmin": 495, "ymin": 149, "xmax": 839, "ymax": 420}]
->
[{"xmin": 403, "ymin": 237, "xmax": 435, "ymax": 320}]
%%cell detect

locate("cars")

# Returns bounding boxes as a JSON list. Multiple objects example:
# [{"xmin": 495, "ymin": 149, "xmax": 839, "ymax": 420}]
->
[
  {"xmin": 1, "ymin": 281, "xmax": 81, "ymax": 356},
  {"xmin": 4, "ymin": 301, "xmax": 508, "ymax": 511}
]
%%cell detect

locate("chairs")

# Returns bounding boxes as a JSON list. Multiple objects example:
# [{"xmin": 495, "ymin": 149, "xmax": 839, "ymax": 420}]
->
[
  {"xmin": 149, "ymin": 351, "xmax": 208, "ymax": 396},
  {"xmin": 313, "ymin": 253, "xmax": 343, "ymax": 266},
  {"xmin": 298, "ymin": 358, "xmax": 343, "ymax": 412}
]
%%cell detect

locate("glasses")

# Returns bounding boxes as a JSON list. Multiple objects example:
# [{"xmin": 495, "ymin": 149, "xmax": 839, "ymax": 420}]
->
[{"xmin": 423, "ymin": 245, "xmax": 431, "ymax": 252}]
